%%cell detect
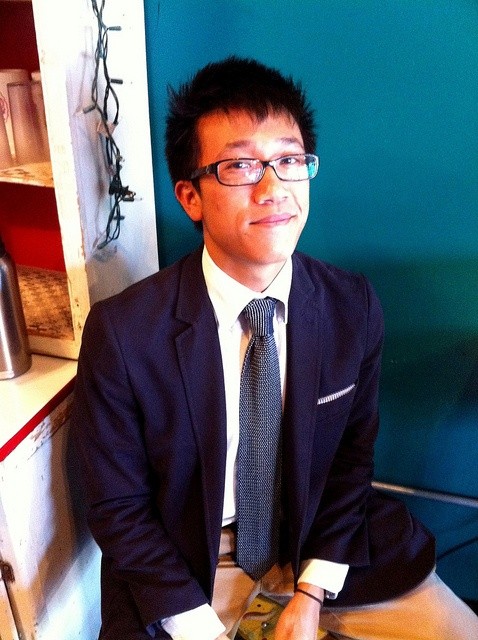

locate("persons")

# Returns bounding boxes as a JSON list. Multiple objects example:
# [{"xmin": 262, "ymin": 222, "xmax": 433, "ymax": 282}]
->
[{"xmin": 70, "ymin": 55, "xmax": 477, "ymax": 640}]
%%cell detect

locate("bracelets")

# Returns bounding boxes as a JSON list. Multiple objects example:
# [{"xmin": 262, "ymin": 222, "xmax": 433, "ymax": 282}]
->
[{"xmin": 294, "ymin": 587, "xmax": 323, "ymax": 606}]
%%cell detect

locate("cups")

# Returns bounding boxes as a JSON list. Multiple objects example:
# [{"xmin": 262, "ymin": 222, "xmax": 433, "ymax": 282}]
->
[
  {"xmin": 0, "ymin": 104, "xmax": 14, "ymax": 170},
  {"xmin": 8, "ymin": 82, "xmax": 50, "ymax": 165},
  {"xmin": 30, "ymin": 71, "xmax": 53, "ymax": 154},
  {"xmin": 1, "ymin": 67, "xmax": 29, "ymax": 159}
]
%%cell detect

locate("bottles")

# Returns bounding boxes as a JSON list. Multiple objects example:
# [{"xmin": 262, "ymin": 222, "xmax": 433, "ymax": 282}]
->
[{"xmin": 0, "ymin": 238, "xmax": 31, "ymax": 381}]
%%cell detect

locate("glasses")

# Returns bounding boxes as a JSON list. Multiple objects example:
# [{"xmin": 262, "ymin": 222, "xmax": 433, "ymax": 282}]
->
[
  {"xmin": 189, "ymin": 153, "xmax": 320, "ymax": 186},
  {"xmin": 236, "ymin": 296, "xmax": 284, "ymax": 582}
]
